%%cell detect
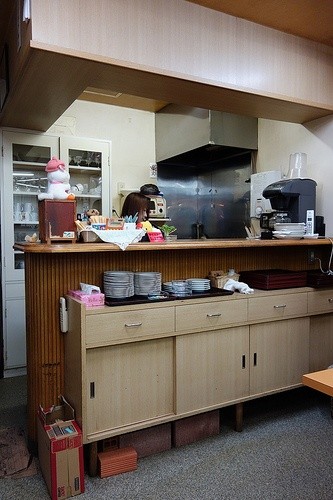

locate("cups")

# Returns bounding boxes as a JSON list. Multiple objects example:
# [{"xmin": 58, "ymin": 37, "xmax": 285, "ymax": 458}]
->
[
  {"xmin": 91, "ymin": 223, "xmax": 106, "ymax": 230},
  {"xmin": 13, "ymin": 202, "xmax": 38, "ymax": 222},
  {"xmin": 124, "ymin": 223, "xmax": 136, "ymax": 231}
]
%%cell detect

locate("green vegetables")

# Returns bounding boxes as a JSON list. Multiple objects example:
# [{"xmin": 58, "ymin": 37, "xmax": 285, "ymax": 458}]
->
[{"xmin": 161, "ymin": 224, "xmax": 175, "ymax": 236}]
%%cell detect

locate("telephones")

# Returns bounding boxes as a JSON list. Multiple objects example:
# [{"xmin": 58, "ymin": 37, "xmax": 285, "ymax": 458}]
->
[{"xmin": 303, "ymin": 210, "xmax": 319, "ymax": 239}]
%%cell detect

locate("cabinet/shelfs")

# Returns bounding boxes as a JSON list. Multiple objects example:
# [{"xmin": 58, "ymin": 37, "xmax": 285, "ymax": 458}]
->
[
  {"xmin": 0, "ymin": 127, "xmax": 112, "ymax": 378},
  {"xmin": 69, "ymin": 288, "xmax": 333, "ymax": 477}
]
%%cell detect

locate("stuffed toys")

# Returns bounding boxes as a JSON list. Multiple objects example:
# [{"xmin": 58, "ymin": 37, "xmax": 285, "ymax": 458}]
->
[
  {"xmin": 37, "ymin": 157, "xmax": 76, "ymax": 200},
  {"xmin": 86, "ymin": 209, "xmax": 98, "ymax": 225}
]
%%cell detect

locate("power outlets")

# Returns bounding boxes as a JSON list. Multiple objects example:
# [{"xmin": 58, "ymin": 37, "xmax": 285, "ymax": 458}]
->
[{"xmin": 112, "ymin": 205, "xmax": 115, "ymax": 216}]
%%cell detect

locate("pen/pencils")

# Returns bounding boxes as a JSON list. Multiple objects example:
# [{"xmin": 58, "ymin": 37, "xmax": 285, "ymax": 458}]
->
[{"xmin": 123, "ymin": 212, "xmax": 139, "ymax": 223}]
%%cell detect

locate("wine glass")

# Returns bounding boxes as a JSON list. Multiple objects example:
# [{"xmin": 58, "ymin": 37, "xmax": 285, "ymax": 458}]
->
[{"xmin": 69, "ymin": 151, "xmax": 100, "ymax": 167}]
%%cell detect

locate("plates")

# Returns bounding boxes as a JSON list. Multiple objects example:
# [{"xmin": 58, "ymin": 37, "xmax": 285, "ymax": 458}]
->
[
  {"xmin": 272, "ymin": 222, "xmax": 306, "ymax": 240},
  {"xmin": 104, "ymin": 270, "xmax": 211, "ymax": 298}
]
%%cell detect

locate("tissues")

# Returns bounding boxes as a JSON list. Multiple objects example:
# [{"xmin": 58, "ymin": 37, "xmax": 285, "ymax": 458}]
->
[{"xmin": 67, "ymin": 282, "xmax": 104, "ymax": 307}]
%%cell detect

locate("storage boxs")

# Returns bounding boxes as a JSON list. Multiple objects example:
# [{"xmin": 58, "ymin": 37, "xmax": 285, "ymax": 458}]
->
[
  {"xmin": 38, "ymin": 199, "xmax": 77, "ymax": 245},
  {"xmin": 37, "ymin": 395, "xmax": 86, "ymax": 500},
  {"xmin": 97, "ymin": 449, "xmax": 138, "ymax": 479}
]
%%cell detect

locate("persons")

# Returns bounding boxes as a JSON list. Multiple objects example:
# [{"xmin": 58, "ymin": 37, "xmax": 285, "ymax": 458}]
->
[{"xmin": 117, "ymin": 192, "xmax": 151, "ymax": 242}]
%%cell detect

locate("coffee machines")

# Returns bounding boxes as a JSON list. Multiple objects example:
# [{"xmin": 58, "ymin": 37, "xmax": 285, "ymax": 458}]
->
[{"xmin": 259, "ymin": 178, "xmax": 317, "ymax": 240}]
